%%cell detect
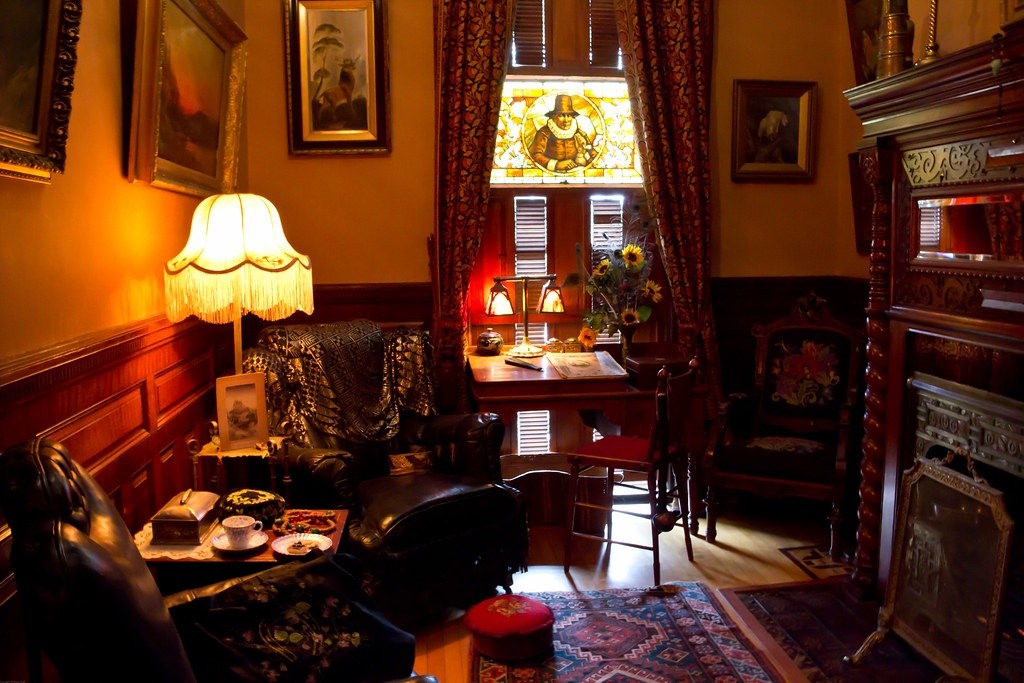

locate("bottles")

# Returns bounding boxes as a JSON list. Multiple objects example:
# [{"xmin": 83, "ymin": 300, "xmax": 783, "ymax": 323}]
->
[{"xmin": 476, "ymin": 328, "xmax": 504, "ymax": 355}]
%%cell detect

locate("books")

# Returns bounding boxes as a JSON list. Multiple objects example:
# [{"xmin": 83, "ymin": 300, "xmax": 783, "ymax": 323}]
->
[{"xmin": 546, "ymin": 351, "xmax": 629, "ymax": 379}]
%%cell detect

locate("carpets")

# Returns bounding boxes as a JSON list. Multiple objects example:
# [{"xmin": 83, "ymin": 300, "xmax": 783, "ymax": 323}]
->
[
  {"xmin": 469, "ymin": 581, "xmax": 783, "ymax": 683},
  {"xmin": 716, "ymin": 574, "xmax": 944, "ymax": 683}
]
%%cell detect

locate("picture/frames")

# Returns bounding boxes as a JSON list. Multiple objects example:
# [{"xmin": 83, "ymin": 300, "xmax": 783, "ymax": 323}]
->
[
  {"xmin": 128, "ymin": 0, "xmax": 249, "ymax": 200},
  {"xmin": 283, "ymin": 0, "xmax": 392, "ymax": 157},
  {"xmin": 731, "ymin": 79, "xmax": 818, "ymax": 183},
  {"xmin": 845, "ymin": 0, "xmax": 907, "ymax": 87},
  {"xmin": 849, "ymin": 152, "xmax": 871, "ymax": 256},
  {"xmin": 216, "ymin": 372, "xmax": 269, "ymax": 451},
  {"xmin": 0, "ymin": 0, "xmax": 82, "ymax": 186}
]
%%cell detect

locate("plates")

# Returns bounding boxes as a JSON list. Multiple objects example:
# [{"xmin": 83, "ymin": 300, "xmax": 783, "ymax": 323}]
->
[
  {"xmin": 210, "ymin": 529, "xmax": 269, "ymax": 551},
  {"xmin": 271, "ymin": 533, "xmax": 333, "ymax": 556}
]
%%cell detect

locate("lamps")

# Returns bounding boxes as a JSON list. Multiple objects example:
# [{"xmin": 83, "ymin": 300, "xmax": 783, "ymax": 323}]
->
[
  {"xmin": 163, "ymin": 185, "xmax": 314, "ymax": 375},
  {"xmin": 485, "ymin": 274, "xmax": 564, "ymax": 357}
]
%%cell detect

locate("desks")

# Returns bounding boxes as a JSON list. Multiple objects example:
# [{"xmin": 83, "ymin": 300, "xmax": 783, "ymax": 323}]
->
[
  {"xmin": 134, "ymin": 509, "xmax": 348, "ymax": 562},
  {"xmin": 467, "ymin": 344, "xmax": 630, "ymax": 482}
]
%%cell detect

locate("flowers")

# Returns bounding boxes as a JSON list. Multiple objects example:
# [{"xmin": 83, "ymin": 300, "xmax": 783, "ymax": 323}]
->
[{"xmin": 577, "ymin": 243, "xmax": 663, "ymax": 348}]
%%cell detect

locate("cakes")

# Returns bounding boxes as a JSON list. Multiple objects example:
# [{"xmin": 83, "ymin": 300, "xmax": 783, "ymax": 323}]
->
[{"xmin": 214, "ymin": 488, "xmax": 285, "ymax": 527}]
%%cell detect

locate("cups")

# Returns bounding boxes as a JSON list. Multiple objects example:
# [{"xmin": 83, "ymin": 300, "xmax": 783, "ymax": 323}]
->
[{"xmin": 222, "ymin": 515, "xmax": 263, "ymax": 548}]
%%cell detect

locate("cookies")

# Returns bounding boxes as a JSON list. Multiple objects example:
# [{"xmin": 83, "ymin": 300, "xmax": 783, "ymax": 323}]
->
[{"xmin": 287, "ymin": 541, "xmax": 308, "ymax": 554}]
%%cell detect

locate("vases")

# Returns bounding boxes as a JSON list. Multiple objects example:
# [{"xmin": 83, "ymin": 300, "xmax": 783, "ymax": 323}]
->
[{"xmin": 619, "ymin": 325, "xmax": 636, "ymax": 371}]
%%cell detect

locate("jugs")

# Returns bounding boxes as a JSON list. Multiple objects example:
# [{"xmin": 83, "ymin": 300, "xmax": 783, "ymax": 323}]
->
[{"xmin": 875, "ymin": 12, "xmax": 915, "ymax": 80}]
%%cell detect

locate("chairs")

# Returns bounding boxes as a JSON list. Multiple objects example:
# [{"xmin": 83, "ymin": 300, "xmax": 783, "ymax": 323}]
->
[
  {"xmin": 567, "ymin": 355, "xmax": 698, "ymax": 587},
  {"xmin": 699, "ymin": 290, "xmax": 867, "ymax": 563},
  {"xmin": 243, "ymin": 321, "xmax": 530, "ymax": 595},
  {"xmin": 0, "ymin": 437, "xmax": 438, "ymax": 683}
]
